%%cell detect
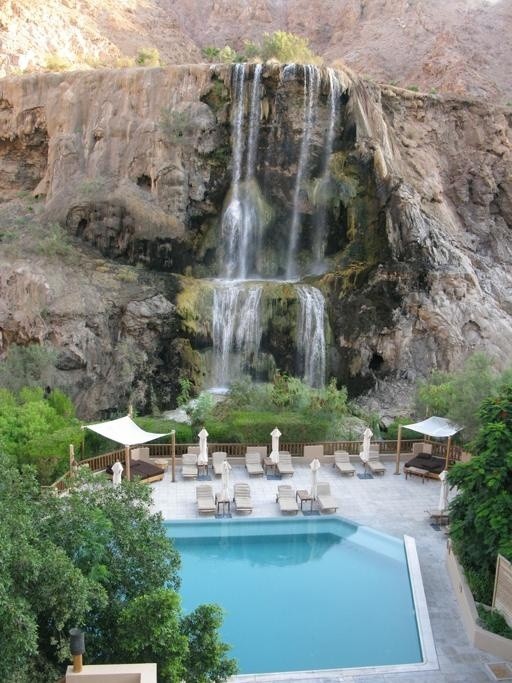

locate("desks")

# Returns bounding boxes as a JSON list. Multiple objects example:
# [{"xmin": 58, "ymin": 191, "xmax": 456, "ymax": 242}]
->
[
  {"xmin": 406, "ymin": 467, "xmax": 429, "ymax": 484},
  {"xmin": 154, "ymin": 459, "xmax": 168, "ymax": 471}
]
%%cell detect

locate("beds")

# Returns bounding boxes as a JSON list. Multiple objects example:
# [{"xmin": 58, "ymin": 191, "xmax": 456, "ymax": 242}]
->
[
  {"xmin": 404, "ymin": 452, "xmax": 454, "ymax": 480},
  {"xmin": 106, "ymin": 459, "xmax": 164, "ymax": 485}
]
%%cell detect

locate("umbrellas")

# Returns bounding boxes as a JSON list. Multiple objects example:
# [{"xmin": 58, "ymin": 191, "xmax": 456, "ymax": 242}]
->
[
  {"xmin": 308, "ymin": 457, "xmax": 320, "ymax": 511},
  {"xmin": 196, "ymin": 427, "xmax": 210, "ymax": 474},
  {"xmin": 268, "ymin": 425, "xmax": 282, "ymax": 476},
  {"xmin": 220, "ymin": 459, "xmax": 233, "ymax": 514},
  {"xmin": 110, "ymin": 459, "xmax": 124, "ymax": 487},
  {"xmin": 358, "ymin": 428, "xmax": 374, "ymax": 475},
  {"xmin": 439, "ymin": 468, "xmax": 451, "ymax": 523}
]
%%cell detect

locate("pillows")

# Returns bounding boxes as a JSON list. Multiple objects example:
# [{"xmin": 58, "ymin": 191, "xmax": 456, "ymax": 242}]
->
[
  {"xmin": 417, "ymin": 453, "xmax": 431, "ymax": 458},
  {"xmin": 121, "ymin": 460, "xmax": 139, "ymax": 468},
  {"xmin": 423, "ymin": 462, "xmax": 439, "ymax": 468}
]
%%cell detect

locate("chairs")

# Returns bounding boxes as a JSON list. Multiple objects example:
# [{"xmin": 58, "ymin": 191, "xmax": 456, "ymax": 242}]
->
[
  {"xmin": 334, "ymin": 449, "xmax": 387, "ymax": 476},
  {"xmin": 195, "ymin": 481, "xmax": 339, "ymax": 515},
  {"xmin": 180, "ymin": 451, "xmax": 295, "ymax": 480}
]
